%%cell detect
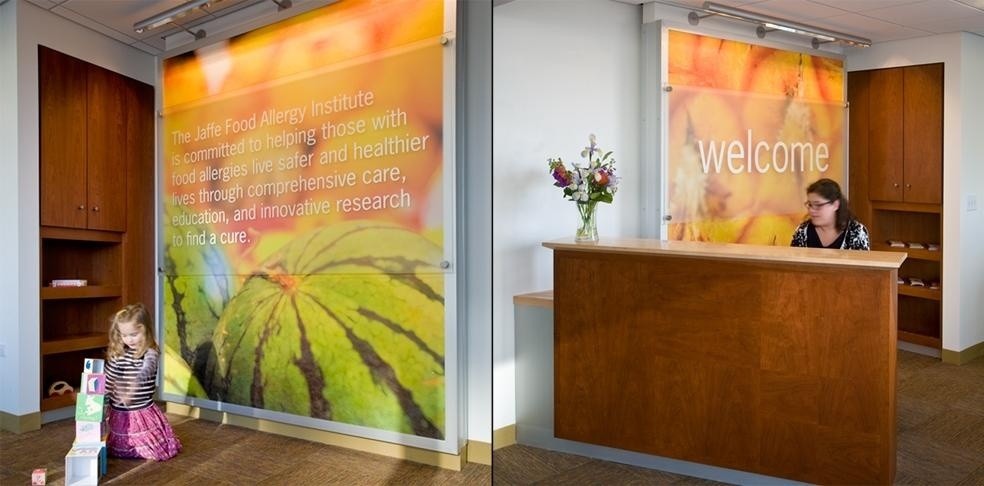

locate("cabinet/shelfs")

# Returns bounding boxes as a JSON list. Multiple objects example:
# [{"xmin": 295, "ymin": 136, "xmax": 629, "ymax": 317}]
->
[
  {"xmin": 37, "ymin": 41, "xmax": 156, "ymax": 425},
  {"xmin": 848, "ymin": 62, "xmax": 942, "ymax": 348}
]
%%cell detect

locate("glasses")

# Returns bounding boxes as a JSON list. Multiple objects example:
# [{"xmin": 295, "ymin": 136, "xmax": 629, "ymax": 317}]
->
[{"xmin": 804, "ymin": 200, "xmax": 833, "ymax": 211}]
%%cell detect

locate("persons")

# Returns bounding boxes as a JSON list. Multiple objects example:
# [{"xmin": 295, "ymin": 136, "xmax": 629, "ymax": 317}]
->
[
  {"xmin": 791, "ymin": 177, "xmax": 870, "ymax": 250},
  {"xmin": 102, "ymin": 302, "xmax": 183, "ymax": 463}
]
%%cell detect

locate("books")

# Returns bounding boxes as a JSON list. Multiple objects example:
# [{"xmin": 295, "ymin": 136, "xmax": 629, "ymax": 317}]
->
[
  {"xmin": 888, "ymin": 239, "xmax": 904, "ymax": 248},
  {"xmin": 929, "ymin": 280, "xmax": 940, "ymax": 289},
  {"xmin": 927, "ymin": 242, "xmax": 939, "ymax": 250},
  {"xmin": 907, "ymin": 242, "xmax": 924, "ymax": 248},
  {"xmin": 897, "ymin": 276, "xmax": 904, "ymax": 285},
  {"xmin": 48, "ymin": 279, "xmax": 89, "ymax": 287},
  {"xmin": 908, "ymin": 277, "xmax": 925, "ymax": 287}
]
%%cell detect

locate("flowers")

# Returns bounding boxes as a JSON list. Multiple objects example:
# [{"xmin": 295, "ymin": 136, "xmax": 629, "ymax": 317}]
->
[{"xmin": 546, "ymin": 131, "xmax": 620, "ymax": 228}]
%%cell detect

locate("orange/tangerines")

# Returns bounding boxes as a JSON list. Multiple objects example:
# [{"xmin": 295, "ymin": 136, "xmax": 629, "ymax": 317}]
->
[{"xmin": 162, "ymin": 2, "xmax": 443, "ymax": 295}]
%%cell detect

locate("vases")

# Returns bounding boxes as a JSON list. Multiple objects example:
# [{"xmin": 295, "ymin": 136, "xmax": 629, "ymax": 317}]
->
[{"xmin": 573, "ymin": 201, "xmax": 598, "ymax": 243}]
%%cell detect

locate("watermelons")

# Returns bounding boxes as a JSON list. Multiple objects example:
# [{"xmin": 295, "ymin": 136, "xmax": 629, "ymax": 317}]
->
[
  {"xmin": 204, "ymin": 219, "xmax": 445, "ymax": 440},
  {"xmin": 162, "ymin": 193, "xmax": 241, "ymax": 384},
  {"xmin": 163, "ymin": 345, "xmax": 213, "ymax": 400}
]
